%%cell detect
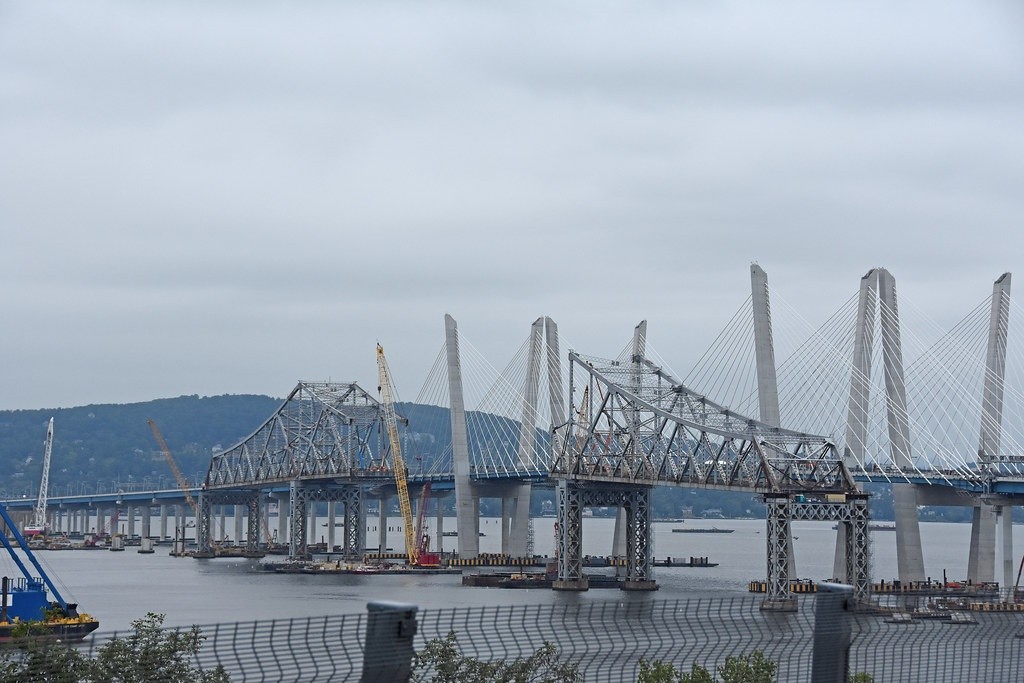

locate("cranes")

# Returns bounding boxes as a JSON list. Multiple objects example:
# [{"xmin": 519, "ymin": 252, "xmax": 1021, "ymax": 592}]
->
[
  {"xmin": 22, "ymin": 416, "xmax": 70, "ymax": 543},
  {"xmin": 375, "ymin": 342, "xmax": 445, "ymax": 569},
  {"xmin": 76, "ymin": 509, "xmax": 122, "ymax": 547},
  {"xmin": 145, "ymin": 418, "xmax": 243, "ymax": 557}
]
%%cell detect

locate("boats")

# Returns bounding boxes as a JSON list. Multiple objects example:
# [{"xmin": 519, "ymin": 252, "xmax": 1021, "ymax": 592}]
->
[
  {"xmin": 649, "ymin": 556, "xmax": 719, "ymax": 568},
  {"xmin": 650, "ymin": 518, "xmax": 685, "ymax": 523},
  {"xmin": 831, "ymin": 523, "xmax": 895, "ymax": 531},
  {"xmin": 883, "ymin": 599, "xmax": 980, "ymax": 625},
  {"xmin": 0, "ymin": 496, "xmax": 101, "ymax": 646},
  {"xmin": 436, "ymin": 531, "xmax": 486, "ymax": 537},
  {"xmin": 672, "ymin": 530, "xmax": 735, "ymax": 533},
  {"xmin": 322, "ymin": 523, "xmax": 344, "ymax": 527}
]
